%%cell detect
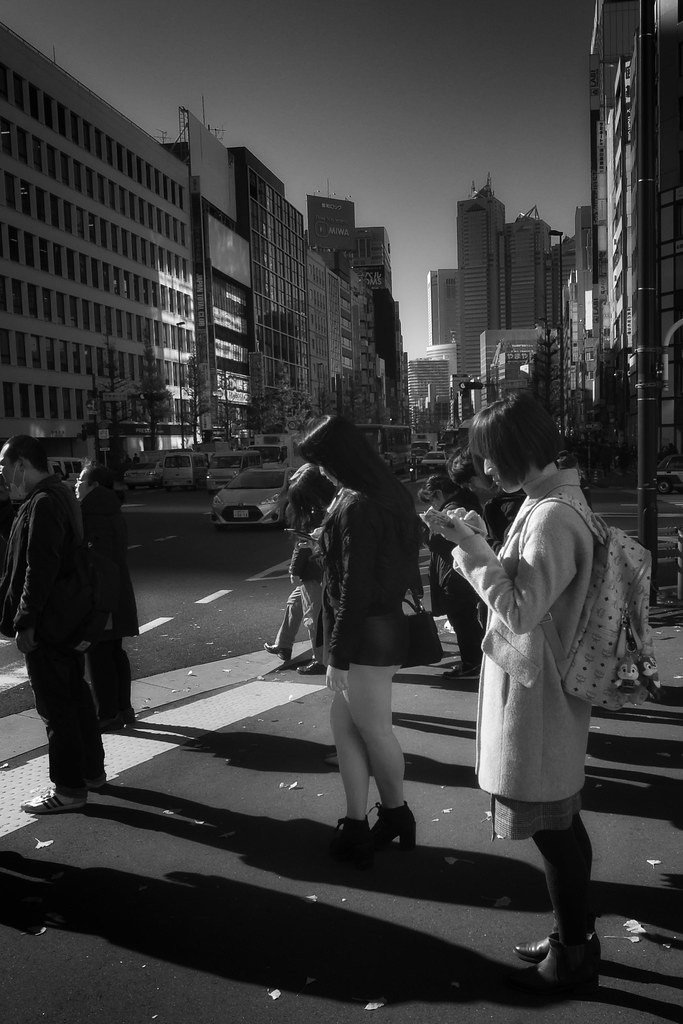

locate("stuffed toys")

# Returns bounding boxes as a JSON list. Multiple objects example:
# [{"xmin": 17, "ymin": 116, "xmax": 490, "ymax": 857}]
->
[{"xmin": 612, "ymin": 655, "xmax": 666, "ymax": 705}]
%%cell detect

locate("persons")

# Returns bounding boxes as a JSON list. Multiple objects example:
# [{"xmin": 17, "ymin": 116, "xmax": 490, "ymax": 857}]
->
[
  {"xmin": 657, "ymin": 443, "xmax": 678, "ymax": 462},
  {"xmin": 0, "ymin": 434, "xmax": 106, "ymax": 813},
  {"xmin": 614, "ymin": 441, "xmax": 637, "ymax": 470},
  {"xmin": 117, "ymin": 453, "xmax": 139, "ymax": 472},
  {"xmin": 438, "ymin": 392, "xmax": 595, "ymax": 995},
  {"xmin": 0, "ymin": 475, "xmax": 16, "ymax": 542},
  {"xmin": 264, "ymin": 465, "xmax": 340, "ymax": 674},
  {"xmin": 418, "ymin": 447, "xmax": 527, "ymax": 679},
  {"xmin": 298, "ymin": 415, "xmax": 416, "ymax": 871},
  {"xmin": 73, "ymin": 464, "xmax": 140, "ymax": 729}
]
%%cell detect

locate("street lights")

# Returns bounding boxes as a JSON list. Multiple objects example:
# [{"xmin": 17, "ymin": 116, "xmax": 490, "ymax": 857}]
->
[
  {"xmin": 540, "ymin": 317, "xmax": 547, "ymax": 352},
  {"xmin": 178, "ymin": 321, "xmax": 185, "ymax": 449},
  {"xmin": 317, "ymin": 362, "xmax": 323, "ymax": 410},
  {"xmin": 549, "ymin": 230, "xmax": 565, "ymax": 438}
]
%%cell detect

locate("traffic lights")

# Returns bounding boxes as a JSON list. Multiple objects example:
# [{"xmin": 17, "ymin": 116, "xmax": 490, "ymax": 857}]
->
[
  {"xmin": 139, "ymin": 392, "xmax": 165, "ymax": 402},
  {"xmin": 458, "ymin": 382, "xmax": 482, "ymax": 390}
]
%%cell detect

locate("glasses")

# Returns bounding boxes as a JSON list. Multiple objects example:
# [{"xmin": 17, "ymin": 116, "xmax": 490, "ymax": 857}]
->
[{"xmin": 76, "ymin": 478, "xmax": 89, "ymax": 484}]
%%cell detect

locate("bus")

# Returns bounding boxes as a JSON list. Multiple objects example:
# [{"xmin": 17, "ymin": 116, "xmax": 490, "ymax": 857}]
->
[
  {"xmin": 439, "ymin": 428, "xmax": 468, "ymax": 455},
  {"xmin": 356, "ymin": 424, "xmax": 412, "ymax": 474}
]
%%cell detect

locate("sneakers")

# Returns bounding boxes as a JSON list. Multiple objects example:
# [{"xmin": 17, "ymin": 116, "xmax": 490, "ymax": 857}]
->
[
  {"xmin": 83, "ymin": 773, "xmax": 106, "ymax": 788},
  {"xmin": 21, "ymin": 790, "xmax": 87, "ymax": 813},
  {"xmin": 444, "ymin": 663, "xmax": 480, "ymax": 680}
]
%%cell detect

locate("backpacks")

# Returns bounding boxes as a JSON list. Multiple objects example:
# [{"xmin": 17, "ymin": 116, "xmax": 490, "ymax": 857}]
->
[
  {"xmin": 25, "ymin": 488, "xmax": 119, "ymax": 657},
  {"xmin": 519, "ymin": 492, "xmax": 662, "ymax": 710}
]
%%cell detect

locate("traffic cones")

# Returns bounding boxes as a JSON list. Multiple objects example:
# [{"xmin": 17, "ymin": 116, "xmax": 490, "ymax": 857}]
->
[{"xmin": 593, "ymin": 470, "xmax": 598, "ymax": 483}]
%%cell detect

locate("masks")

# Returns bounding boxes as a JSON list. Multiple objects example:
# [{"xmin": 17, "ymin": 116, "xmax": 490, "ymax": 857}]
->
[{"xmin": 10, "ymin": 466, "xmax": 27, "ymax": 501}]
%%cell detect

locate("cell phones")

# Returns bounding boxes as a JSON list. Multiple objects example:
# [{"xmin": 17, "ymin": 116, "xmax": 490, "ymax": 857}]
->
[{"xmin": 284, "ymin": 528, "xmax": 316, "ymax": 543}]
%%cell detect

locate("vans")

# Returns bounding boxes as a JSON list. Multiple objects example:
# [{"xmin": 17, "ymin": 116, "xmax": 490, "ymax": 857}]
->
[
  {"xmin": 205, "ymin": 451, "xmax": 262, "ymax": 494},
  {"xmin": 163, "ymin": 453, "xmax": 210, "ymax": 492},
  {"xmin": 12, "ymin": 457, "xmax": 94, "ymax": 505}
]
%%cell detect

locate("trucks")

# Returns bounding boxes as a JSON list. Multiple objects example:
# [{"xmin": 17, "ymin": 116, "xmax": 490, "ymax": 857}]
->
[
  {"xmin": 245, "ymin": 434, "xmax": 305, "ymax": 469},
  {"xmin": 412, "ymin": 433, "xmax": 437, "ymax": 462}
]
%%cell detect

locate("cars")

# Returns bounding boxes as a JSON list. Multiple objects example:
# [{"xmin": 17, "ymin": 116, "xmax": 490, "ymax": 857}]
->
[
  {"xmin": 421, "ymin": 451, "xmax": 448, "ymax": 471},
  {"xmin": 123, "ymin": 461, "xmax": 164, "ymax": 491},
  {"xmin": 657, "ymin": 453, "xmax": 683, "ymax": 493},
  {"xmin": 210, "ymin": 467, "xmax": 299, "ymax": 532}
]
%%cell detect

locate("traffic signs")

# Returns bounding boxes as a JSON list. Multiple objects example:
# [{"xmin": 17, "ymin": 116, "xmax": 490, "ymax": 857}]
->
[{"xmin": 585, "ymin": 423, "xmax": 603, "ymax": 429}]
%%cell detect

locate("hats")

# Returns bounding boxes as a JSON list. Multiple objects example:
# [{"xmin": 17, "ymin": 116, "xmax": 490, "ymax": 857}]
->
[{"xmin": 559, "ymin": 450, "xmax": 568, "ymax": 456}]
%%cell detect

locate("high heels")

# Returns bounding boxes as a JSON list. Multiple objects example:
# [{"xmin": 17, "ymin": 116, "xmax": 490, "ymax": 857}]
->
[
  {"xmin": 367, "ymin": 801, "xmax": 416, "ymax": 849},
  {"xmin": 329, "ymin": 815, "xmax": 373, "ymax": 858}
]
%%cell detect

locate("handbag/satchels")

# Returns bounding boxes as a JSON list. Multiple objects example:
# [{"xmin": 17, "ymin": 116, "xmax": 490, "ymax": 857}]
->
[
  {"xmin": 582, "ymin": 489, "xmax": 592, "ymax": 508},
  {"xmin": 401, "ymin": 598, "xmax": 443, "ymax": 667}
]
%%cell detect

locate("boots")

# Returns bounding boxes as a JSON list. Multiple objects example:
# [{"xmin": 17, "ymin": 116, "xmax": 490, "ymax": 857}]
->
[
  {"xmin": 513, "ymin": 912, "xmax": 601, "ymax": 963},
  {"xmin": 489, "ymin": 933, "xmax": 600, "ymax": 1003}
]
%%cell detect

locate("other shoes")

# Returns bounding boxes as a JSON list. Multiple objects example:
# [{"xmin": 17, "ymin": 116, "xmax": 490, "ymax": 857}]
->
[{"xmin": 96, "ymin": 706, "xmax": 136, "ymax": 731}]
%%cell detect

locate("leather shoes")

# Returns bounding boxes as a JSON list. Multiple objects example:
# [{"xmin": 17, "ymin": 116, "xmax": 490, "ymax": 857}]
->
[
  {"xmin": 297, "ymin": 663, "xmax": 327, "ymax": 675},
  {"xmin": 264, "ymin": 640, "xmax": 293, "ymax": 659}
]
